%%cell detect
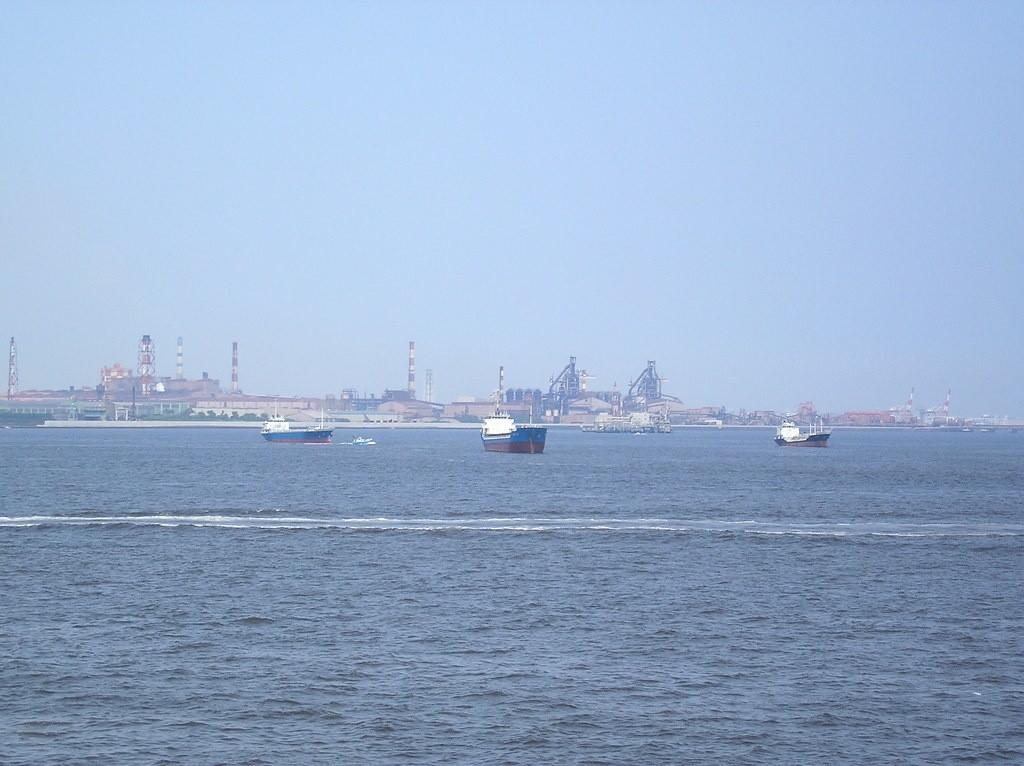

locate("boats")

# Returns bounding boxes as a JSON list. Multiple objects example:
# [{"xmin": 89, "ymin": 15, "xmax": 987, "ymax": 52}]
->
[
  {"xmin": 260, "ymin": 399, "xmax": 334, "ymax": 443},
  {"xmin": 480, "ymin": 389, "xmax": 548, "ymax": 454},
  {"xmin": 352, "ymin": 437, "xmax": 376, "ymax": 445},
  {"xmin": 774, "ymin": 416, "xmax": 830, "ymax": 447}
]
